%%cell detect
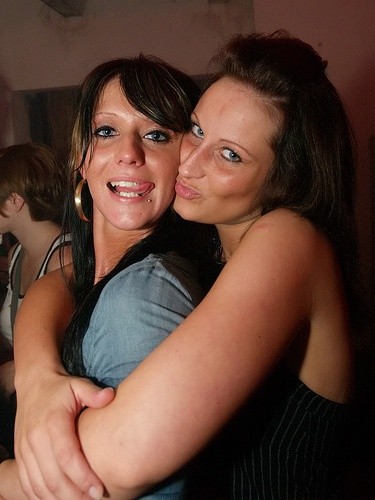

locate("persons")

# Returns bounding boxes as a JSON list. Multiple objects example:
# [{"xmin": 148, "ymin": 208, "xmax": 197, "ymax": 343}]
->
[
  {"xmin": 1, "ymin": 29, "xmax": 364, "ymax": 500},
  {"xmin": 0, "ymin": 51, "xmax": 223, "ymax": 500},
  {"xmin": 0, "ymin": 144, "xmax": 78, "ymax": 404}
]
